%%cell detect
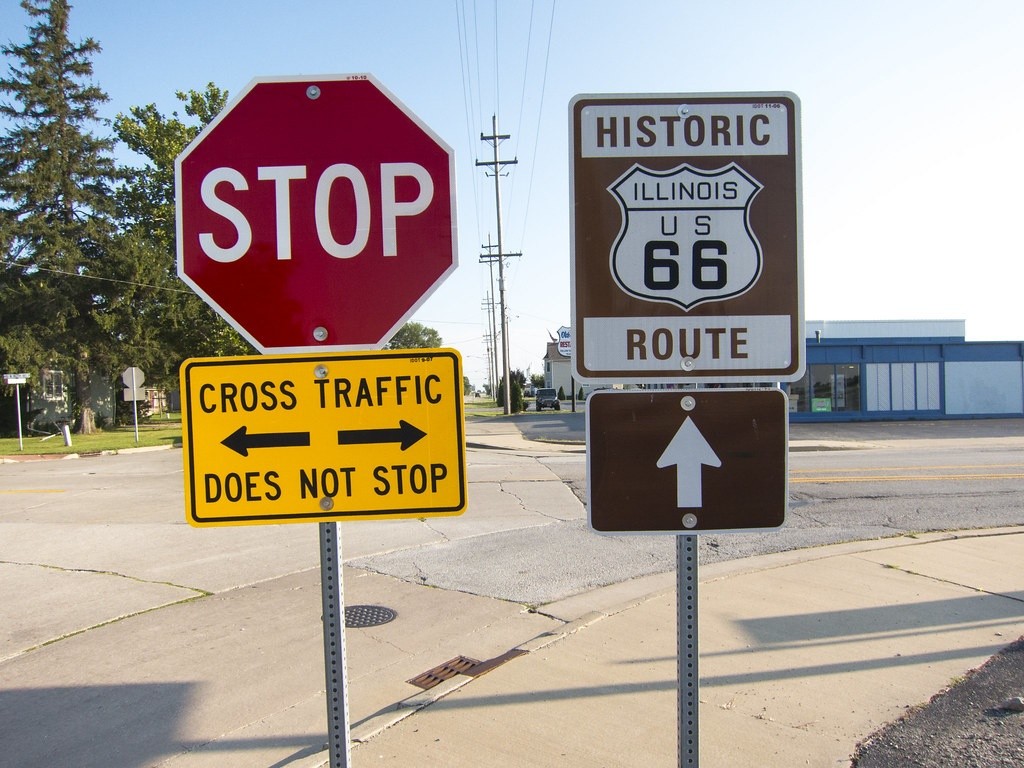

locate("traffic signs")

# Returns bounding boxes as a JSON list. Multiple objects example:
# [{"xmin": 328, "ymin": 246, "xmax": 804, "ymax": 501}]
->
[{"xmin": 184, "ymin": 348, "xmax": 467, "ymax": 524}]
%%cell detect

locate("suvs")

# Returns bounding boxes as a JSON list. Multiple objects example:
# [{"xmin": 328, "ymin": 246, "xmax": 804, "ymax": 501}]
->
[{"xmin": 536, "ymin": 388, "xmax": 560, "ymax": 412}]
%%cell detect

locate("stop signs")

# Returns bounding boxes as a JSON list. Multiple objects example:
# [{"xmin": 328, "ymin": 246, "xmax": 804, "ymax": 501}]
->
[{"xmin": 171, "ymin": 70, "xmax": 456, "ymax": 351}]
